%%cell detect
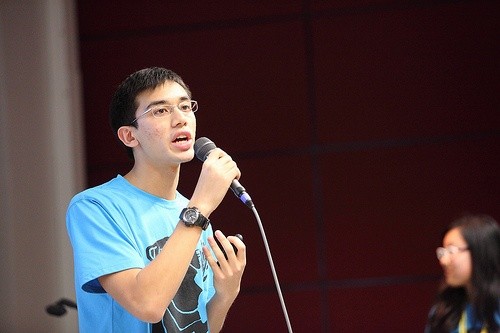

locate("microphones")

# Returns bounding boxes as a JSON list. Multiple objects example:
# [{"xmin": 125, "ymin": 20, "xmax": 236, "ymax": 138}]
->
[{"xmin": 194, "ymin": 137, "xmax": 254, "ymax": 209}]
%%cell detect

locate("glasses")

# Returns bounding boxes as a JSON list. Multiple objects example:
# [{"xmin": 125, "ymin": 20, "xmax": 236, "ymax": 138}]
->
[
  {"xmin": 131, "ymin": 101, "xmax": 198, "ymax": 124},
  {"xmin": 437, "ymin": 247, "xmax": 469, "ymax": 259}
]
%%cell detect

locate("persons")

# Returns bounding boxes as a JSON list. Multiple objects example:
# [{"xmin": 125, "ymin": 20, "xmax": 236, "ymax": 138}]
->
[
  {"xmin": 425, "ymin": 216, "xmax": 500, "ymax": 333},
  {"xmin": 66, "ymin": 67, "xmax": 247, "ymax": 333}
]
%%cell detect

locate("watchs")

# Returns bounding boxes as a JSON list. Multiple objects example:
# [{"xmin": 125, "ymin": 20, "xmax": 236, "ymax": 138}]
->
[{"xmin": 179, "ymin": 207, "xmax": 210, "ymax": 231}]
